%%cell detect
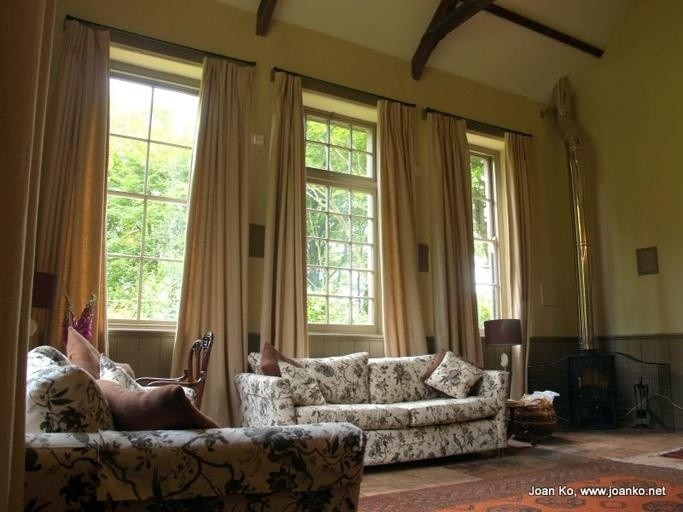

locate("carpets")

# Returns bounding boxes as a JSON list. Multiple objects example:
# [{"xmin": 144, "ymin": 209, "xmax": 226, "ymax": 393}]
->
[
  {"xmin": 659, "ymin": 447, "xmax": 683, "ymax": 459},
  {"xmin": 357, "ymin": 458, "xmax": 683, "ymax": 512}
]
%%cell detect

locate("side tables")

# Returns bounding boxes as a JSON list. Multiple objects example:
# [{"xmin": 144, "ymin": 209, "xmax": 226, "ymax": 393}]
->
[{"xmin": 506, "ymin": 399, "xmax": 539, "ymax": 446}]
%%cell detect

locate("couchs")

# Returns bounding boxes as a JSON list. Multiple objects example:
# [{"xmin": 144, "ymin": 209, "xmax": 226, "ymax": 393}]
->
[
  {"xmin": 233, "ymin": 370, "xmax": 509, "ymax": 466},
  {"xmin": 25, "ymin": 422, "xmax": 366, "ymax": 512}
]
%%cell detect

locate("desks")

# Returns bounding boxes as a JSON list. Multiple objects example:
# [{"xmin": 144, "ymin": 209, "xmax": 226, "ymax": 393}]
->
[{"xmin": 568, "ymin": 354, "xmax": 616, "ymax": 429}]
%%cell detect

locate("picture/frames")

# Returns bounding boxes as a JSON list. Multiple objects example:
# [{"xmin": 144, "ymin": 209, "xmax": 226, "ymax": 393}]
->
[{"xmin": 636, "ymin": 246, "xmax": 659, "ymax": 276}]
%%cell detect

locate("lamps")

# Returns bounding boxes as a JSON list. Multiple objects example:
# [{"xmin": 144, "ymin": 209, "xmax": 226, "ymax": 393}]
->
[
  {"xmin": 32, "ymin": 272, "xmax": 58, "ymax": 309},
  {"xmin": 484, "ymin": 320, "xmax": 522, "ymax": 398}
]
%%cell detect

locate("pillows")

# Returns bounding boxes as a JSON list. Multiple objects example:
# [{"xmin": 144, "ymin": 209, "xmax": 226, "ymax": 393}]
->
[
  {"xmin": 247, "ymin": 342, "xmax": 486, "ymax": 405},
  {"xmin": 26, "ymin": 326, "xmax": 220, "ymax": 433}
]
